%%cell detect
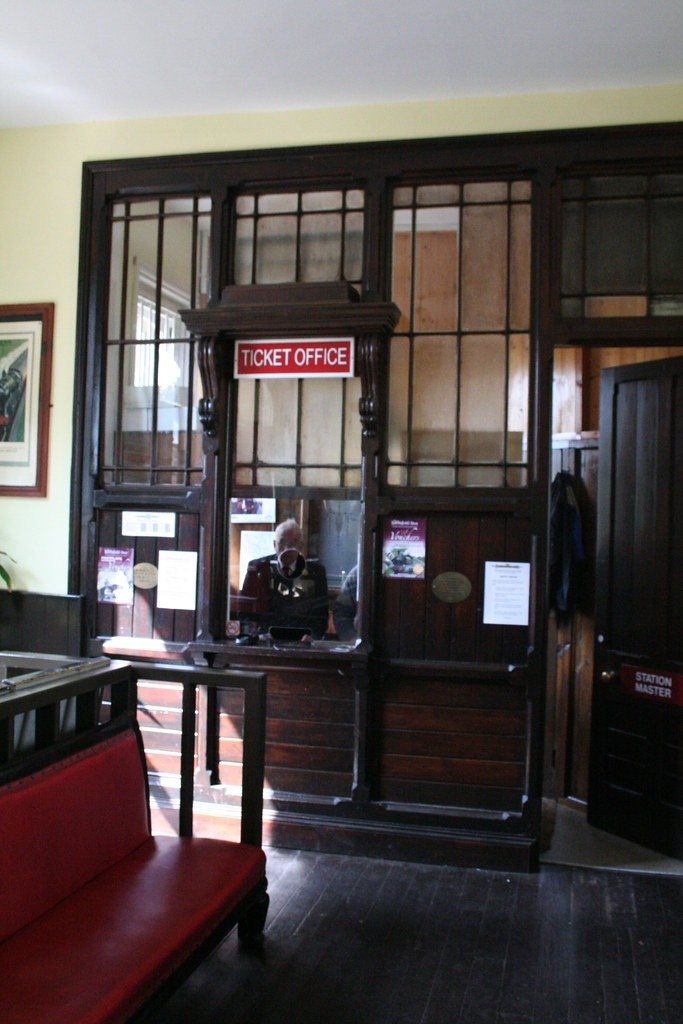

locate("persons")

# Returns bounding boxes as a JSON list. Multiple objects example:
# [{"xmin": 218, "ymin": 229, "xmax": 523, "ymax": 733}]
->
[
  {"xmin": 333, "ymin": 565, "xmax": 358, "ymax": 644},
  {"xmin": 239, "ymin": 519, "xmax": 329, "ymax": 641}
]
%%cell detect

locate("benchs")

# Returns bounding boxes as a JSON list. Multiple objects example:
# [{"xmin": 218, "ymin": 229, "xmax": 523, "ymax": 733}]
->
[{"xmin": 0, "ymin": 713, "xmax": 273, "ymax": 1022}]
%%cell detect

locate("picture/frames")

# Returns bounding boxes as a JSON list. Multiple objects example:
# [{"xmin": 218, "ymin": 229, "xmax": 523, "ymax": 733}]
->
[{"xmin": 1, "ymin": 302, "xmax": 55, "ymax": 498}]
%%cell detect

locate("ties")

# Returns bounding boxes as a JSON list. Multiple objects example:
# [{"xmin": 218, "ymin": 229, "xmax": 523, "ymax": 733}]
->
[{"xmin": 283, "ymin": 566, "xmax": 290, "ymax": 577}]
